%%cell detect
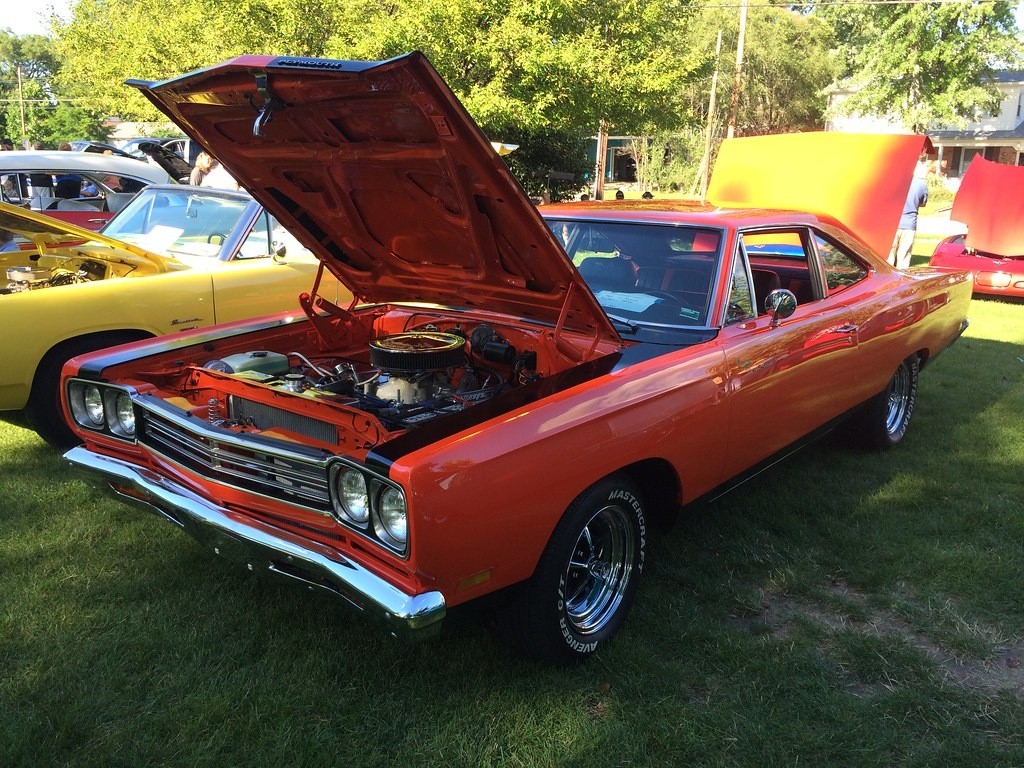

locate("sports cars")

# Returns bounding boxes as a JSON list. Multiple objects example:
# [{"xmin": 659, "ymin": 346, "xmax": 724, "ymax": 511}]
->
[
  {"xmin": 1, "ymin": 142, "xmax": 521, "ymax": 437},
  {"xmin": 927, "ymin": 152, "xmax": 1024, "ymax": 302},
  {"xmin": 58, "ymin": 46, "xmax": 979, "ymax": 659}
]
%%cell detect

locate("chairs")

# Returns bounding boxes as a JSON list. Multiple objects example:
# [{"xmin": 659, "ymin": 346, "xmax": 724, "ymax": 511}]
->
[
  {"xmin": 32, "ymin": 197, "xmax": 64, "ymax": 210},
  {"xmin": 751, "ymin": 268, "xmax": 782, "ymax": 316},
  {"xmin": 57, "ymin": 199, "xmax": 99, "ymax": 211},
  {"xmin": 580, "ymin": 256, "xmax": 636, "ymax": 288}
]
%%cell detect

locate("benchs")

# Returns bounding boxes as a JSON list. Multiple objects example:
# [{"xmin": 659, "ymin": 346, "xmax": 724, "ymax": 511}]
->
[
  {"xmin": 102, "ymin": 193, "xmax": 136, "ymax": 212},
  {"xmin": 635, "ymin": 265, "xmax": 814, "ymax": 312}
]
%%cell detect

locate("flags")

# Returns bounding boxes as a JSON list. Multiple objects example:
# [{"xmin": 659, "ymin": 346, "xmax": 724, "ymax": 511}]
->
[{"xmin": 491, "ymin": 142, "xmax": 519, "ymax": 156}]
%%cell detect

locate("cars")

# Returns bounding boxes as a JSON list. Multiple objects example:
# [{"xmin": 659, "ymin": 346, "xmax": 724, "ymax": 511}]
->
[
  {"xmin": 64, "ymin": 138, "xmax": 209, "ymax": 184},
  {"xmin": 1, "ymin": 151, "xmax": 201, "ymax": 251}
]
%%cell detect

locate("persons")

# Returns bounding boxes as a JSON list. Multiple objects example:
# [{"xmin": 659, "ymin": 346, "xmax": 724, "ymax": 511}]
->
[
  {"xmin": 189, "ymin": 151, "xmax": 239, "ymax": 191},
  {"xmin": 79, "ymin": 183, "xmax": 98, "ymax": 198},
  {"xmin": 642, "ymin": 191, "xmax": 653, "ymax": 199},
  {"xmin": 581, "ymin": 194, "xmax": 589, "ymax": 202},
  {"xmin": 99, "ymin": 150, "xmax": 123, "ymax": 192},
  {"xmin": 0, "ymin": 139, "xmax": 29, "ymax": 198},
  {"xmin": 53, "ymin": 143, "xmax": 84, "ymax": 198},
  {"xmin": 886, "ymin": 176, "xmax": 928, "ymax": 268},
  {"xmin": 616, "ymin": 190, "xmax": 624, "ymax": 200},
  {"xmin": 29, "ymin": 142, "xmax": 55, "ymax": 198}
]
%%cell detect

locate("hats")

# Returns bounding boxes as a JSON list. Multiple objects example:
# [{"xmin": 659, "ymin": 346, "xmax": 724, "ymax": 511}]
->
[
  {"xmin": 1, "ymin": 139, "xmax": 15, "ymax": 146},
  {"xmin": 103, "ymin": 150, "xmax": 113, "ymax": 155}
]
